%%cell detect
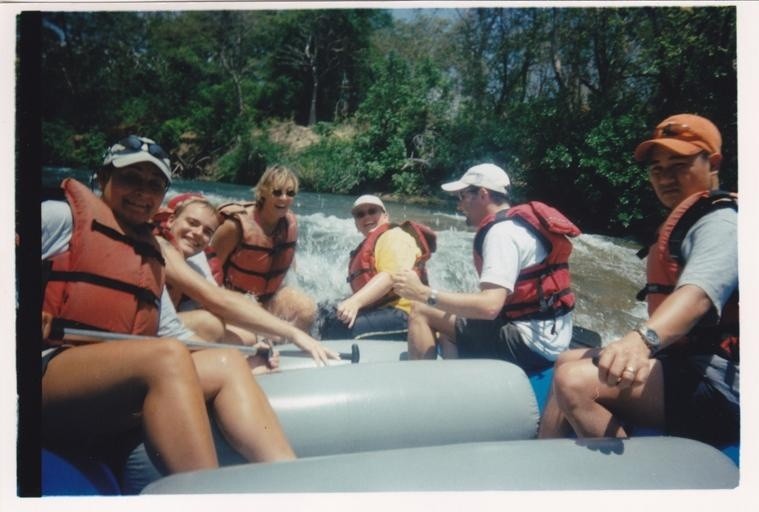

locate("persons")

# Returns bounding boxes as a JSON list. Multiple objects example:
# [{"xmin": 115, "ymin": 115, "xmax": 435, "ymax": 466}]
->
[
  {"xmin": 214, "ymin": 164, "xmax": 316, "ymax": 344},
  {"xmin": 157, "ymin": 193, "xmax": 215, "ymax": 280},
  {"xmin": 324, "ymin": 193, "xmax": 439, "ymax": 339},
  {"xmin": 394, "ymin": 163, "xmax": 578, "ymax": 368},
  {"xmin": 41, "ymin": 133, "xmax": 297, "ymax": 475},
  {"xmin": 537, "ymin": 113, "xmax": 740, "ymax": 438},
  {"xmin": 150, "ymin": 197, "xmax": 341, "ymax": 371}
]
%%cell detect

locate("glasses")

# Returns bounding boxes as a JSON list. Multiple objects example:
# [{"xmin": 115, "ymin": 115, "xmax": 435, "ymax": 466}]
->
[
  {"xmin": 353, "ymin": 207, "xmax": 381, "ymax": 219},
  {"xmin": 114, "ymin": 136, "xmax": 173, "ymax": 163},
  {"xmin": 271, "ymin": 190, "xmax": 296, "ymax": 198}
]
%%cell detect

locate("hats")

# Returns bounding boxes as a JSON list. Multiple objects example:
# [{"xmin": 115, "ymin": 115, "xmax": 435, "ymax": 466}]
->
[
  {"xmin": 634, "ymin": 113, "xmax": 723, "ymax": 163},
  {"xmin": 102, "ymin": 135, "xmax": 173, "ymax": 185},
  {"xmin": 440, "ymin": 164, "xmax": 511, "ymax": 195},
  {"xmin": 353, "ymin": 195, "xmax": 386, "ymax": 213}
]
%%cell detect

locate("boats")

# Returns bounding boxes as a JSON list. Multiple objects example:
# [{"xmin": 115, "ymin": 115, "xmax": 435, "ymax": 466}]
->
[{"xmin": 39, "ymin": 316, "xmax": 737, "ymax": 495}]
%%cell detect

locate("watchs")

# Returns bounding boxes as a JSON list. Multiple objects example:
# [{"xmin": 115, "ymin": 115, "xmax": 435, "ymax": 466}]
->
[
  {"xmin": 635, "ymin": 325, "xmax": 661, "ymax": 357},
  {"xmin": 428, "ymin": 291, "xmax": 437, "ymax": 306}
]
